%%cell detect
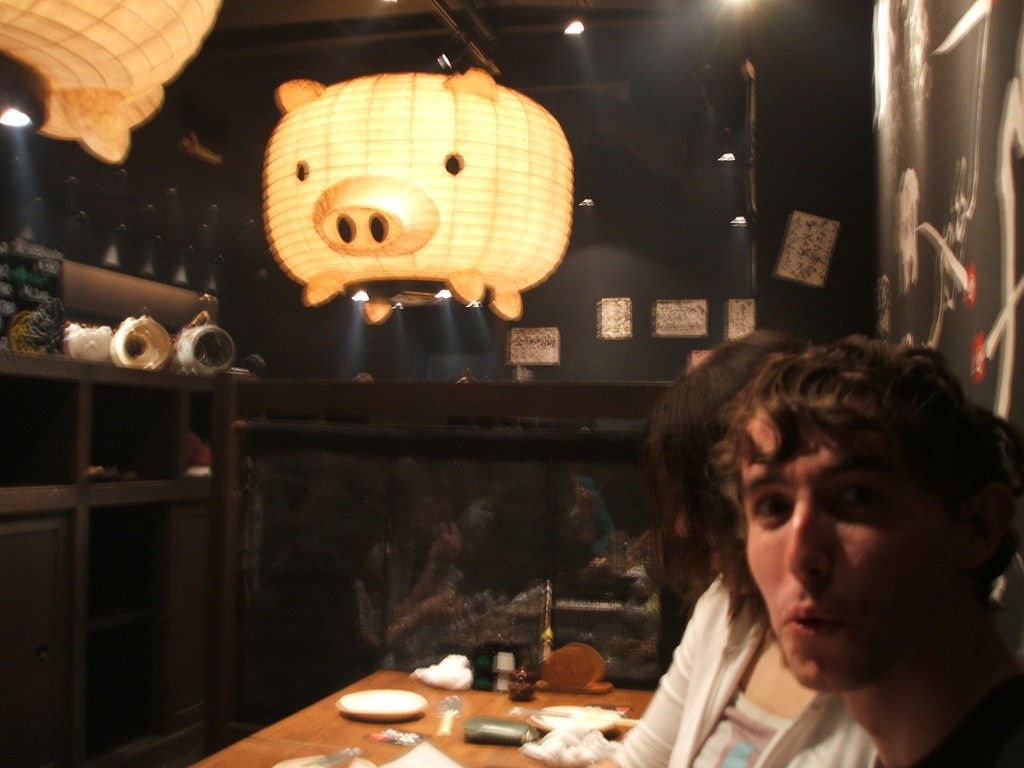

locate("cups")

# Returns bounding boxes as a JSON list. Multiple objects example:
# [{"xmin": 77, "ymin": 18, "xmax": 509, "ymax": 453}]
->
[
  {"xmin": 110, "ymin": 313, "xmax": 172, "ymax": 371},
  {"xmin": 176, "ymin": 310, "xmax": 236, "ymax": 376},
  {"xmin": 616, "ymin": 719, "xmax": 639, "ymax": 734}
]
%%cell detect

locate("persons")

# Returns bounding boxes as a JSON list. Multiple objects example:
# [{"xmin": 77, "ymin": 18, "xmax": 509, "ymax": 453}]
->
[
  {"xmin": 587, "ymin": 330, "xmax": 1024, "ymax": 768},
  {"xmin": 349, "ymin": 458, "xmax": 461, "ymax": 670}
]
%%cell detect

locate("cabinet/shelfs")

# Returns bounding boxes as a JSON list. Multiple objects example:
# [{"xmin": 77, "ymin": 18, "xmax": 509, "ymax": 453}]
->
[{"xmin": 0, "ymin": 350, "xmax": 657, "ymax": 768}]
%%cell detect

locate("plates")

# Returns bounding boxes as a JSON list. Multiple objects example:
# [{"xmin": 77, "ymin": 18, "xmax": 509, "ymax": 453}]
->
[
  {"xmin": 531, "ymin": 705, "xmax": 620, "ymax": 733},
  {"xmin": 272, "ymin": 754, "xmax": 379, "ymax": 768},
  {"xmin": 336, "ymin": 689, "xmax": 428, "ymax": 723}
]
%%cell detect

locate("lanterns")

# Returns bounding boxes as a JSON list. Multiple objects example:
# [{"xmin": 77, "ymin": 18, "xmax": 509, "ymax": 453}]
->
[
  {"xmin": 0, "ymin": 0, "xmax": 223, "ymax": 162},
  {"xmin": 262, "ymin": 68, "xmax": 572, "ymax": 322}
]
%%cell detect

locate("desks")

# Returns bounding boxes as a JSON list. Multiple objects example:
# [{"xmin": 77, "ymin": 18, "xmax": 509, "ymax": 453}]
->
[{"xmin": 189, "ymin": 670, "xmax": 655, "ymax": 768}]
